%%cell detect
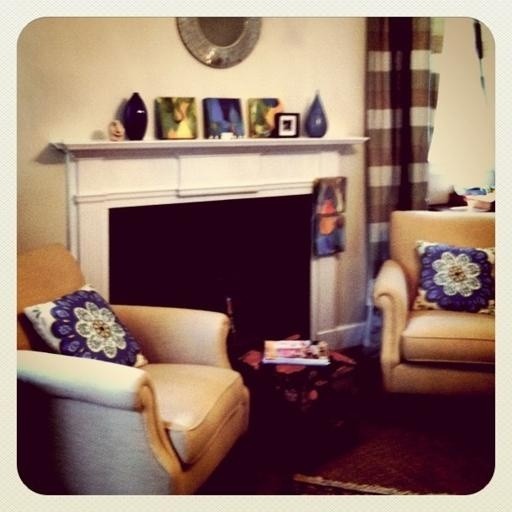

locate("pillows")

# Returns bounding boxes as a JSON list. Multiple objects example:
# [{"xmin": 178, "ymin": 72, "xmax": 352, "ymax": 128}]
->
[
  {"xmin": 412, "ymin": 239, "xmax": 495, "ymax": 316},
  {"xmin": 17, "ymin": 280, "xmax": 148, "ymax": 372}
]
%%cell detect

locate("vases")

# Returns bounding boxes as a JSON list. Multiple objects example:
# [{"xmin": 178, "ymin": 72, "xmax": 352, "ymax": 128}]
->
[
  {"xmin": 123, "ymin": 92, "xmax": 148, "ymax": 140},
  {"xmin": 305, "ymin": 91, "xmax": 328, "ymax": 136}
]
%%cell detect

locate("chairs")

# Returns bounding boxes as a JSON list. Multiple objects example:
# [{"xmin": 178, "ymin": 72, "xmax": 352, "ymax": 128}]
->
[
  {"xmin": 17, "ymin": 244, "xmax": 250, "ymax": 494},
  {"xmin": 370, "ymin": 210, "xmax": 495, "ymax": 394}
]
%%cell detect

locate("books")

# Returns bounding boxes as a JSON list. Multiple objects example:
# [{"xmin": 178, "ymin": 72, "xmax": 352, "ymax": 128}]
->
[{"xmin": 262, "ymin": 340, "xmax": 330, "ymax": 366}]
